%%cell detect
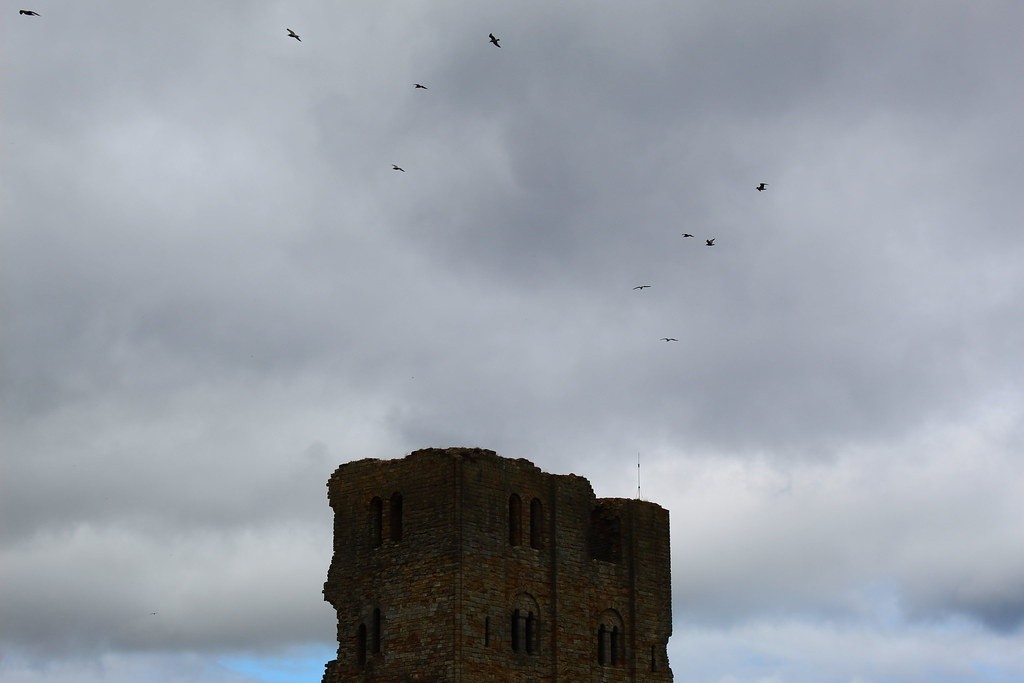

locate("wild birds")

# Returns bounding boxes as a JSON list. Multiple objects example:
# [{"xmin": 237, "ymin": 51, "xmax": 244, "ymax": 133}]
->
[
  {"xmin": 392, "ymin": 164, "xmax": 404, "ymax": 172},
  {"xmin": 489, "ymin": 33, "xmax": 501, "ymax": 47},
  {"xmin": 706, "ymin": 238, "xmax": 715, "ymax": 246},
  {"xmin": 682, "ymin": 233, "xmax": 694, "ymax": 237},
  {"xmin": 757, "ymin": 182, "xmax": 769, "ymax": 192},
  {"xmin": 660, "ymin": 337, "xmax": 678, "ymax": 342},
  {"xmin": 633, "ymin": 285, "xmax": 650, "ymax": 290},
  {"xmin": 19, "ymin": 9, "xmax": 40, "ymax": 16},
  {"xmin": 413, "ymin": 83, "xmax": 427, "ymax": 90},
  {"xmin": 287, "ymin": 28, "xmax": 302, "ymax": 42}
]
